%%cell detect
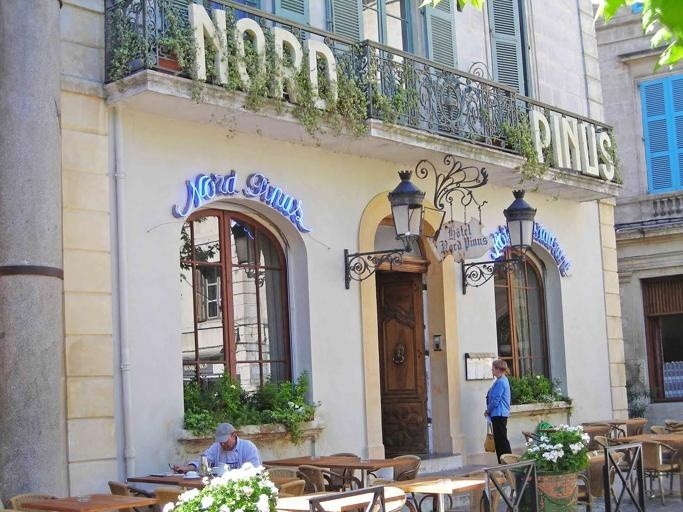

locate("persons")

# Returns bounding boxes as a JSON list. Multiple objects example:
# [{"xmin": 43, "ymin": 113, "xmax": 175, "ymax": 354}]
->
[
  {"xmin": 483, "ymin": 358, "xmax": 514, "ymax": 464},
  {"xmin": 173, "ymin": 422, "xmax": 262, "ymax": 476}
]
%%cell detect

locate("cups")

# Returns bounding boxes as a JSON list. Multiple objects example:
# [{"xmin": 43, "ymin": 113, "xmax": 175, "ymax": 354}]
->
[
  {"xmin": 186, "ymin": 470, "xmax": 198, "ymax": 477},
  {"xmin": 213, "ymin": 466, "xmax": 220, "ymax": 474}
]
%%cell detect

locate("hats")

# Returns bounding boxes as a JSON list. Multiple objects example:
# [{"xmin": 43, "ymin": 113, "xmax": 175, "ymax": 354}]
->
[{"xmin": 215, "ymin": 423, "xmax": 235, "ymax": 443}]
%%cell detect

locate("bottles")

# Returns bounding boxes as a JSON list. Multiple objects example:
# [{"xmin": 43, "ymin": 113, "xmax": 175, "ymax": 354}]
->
[{"xmin": 664, "ymin": 361, "xmax": 683, "ymax": 398}]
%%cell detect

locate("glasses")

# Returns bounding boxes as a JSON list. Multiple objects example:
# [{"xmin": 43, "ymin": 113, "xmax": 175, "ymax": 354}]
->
[{"xmin": 225, "ymin": 449, "xmax": 239, "ymax": 464}]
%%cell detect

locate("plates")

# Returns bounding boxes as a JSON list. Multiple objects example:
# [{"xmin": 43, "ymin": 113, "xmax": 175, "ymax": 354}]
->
[{"xmin": 183, "ymin": 475, "xmax": 201, "ymax": 479}]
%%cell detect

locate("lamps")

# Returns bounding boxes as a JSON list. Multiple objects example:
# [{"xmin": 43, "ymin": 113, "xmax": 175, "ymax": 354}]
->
[
  {"xmin": 345, "ymin": 169, "xmax": 428, "ymax": 291},
  {"xmin": 230, "ymin": 220, "xmax": 271, "ymax": 281},
  {"xmin": 461, "ymin": 188, "xmax": 539, "ymax": 296}
]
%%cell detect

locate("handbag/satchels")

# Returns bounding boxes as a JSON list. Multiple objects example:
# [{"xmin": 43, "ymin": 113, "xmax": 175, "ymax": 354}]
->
[{"xmin": 484, "ymin": 434, "xmax": 496, "ymax": 452}]
